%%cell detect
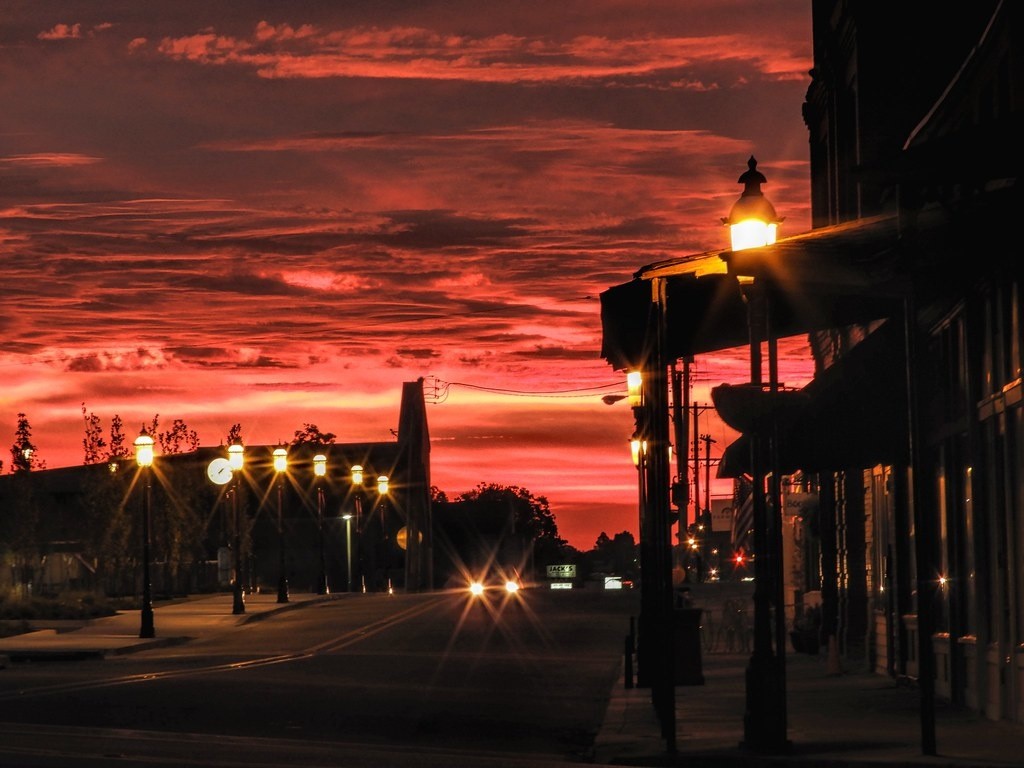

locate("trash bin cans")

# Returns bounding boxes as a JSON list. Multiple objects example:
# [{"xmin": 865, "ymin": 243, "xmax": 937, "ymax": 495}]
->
[{"xmin": 672, "ymin": 604, "xmax": 705, "ymax": 686}]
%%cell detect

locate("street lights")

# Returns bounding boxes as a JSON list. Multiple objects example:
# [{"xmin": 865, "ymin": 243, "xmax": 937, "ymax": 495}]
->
[
  {"xmin": 377, "ymin": 473, "xmax": 394, "ymax": 593},
  {"xmin": 228, "ymin": 438, "xmax": 246, "ymax": 615},
  {"xmin": 273, "ymin": 441, "xmax": 289, "ymax": 603},
  {"xmin": 720, "ymin": 156, "xmax": 790, "ymax": 755},
  {"xmin": 351, "ymin": 460, "xmax": 363, "ymax": 593},
  {"xmin": 314, "ymin": 451, "xmax": 331, "ymax": 595},
  {"xmin": 132, "ymin": 423, "xmax": 155, "ymax": 638},
  {"xmin": 628, "ymin": 371, "xmax": 656, "ymax": 685}
]
